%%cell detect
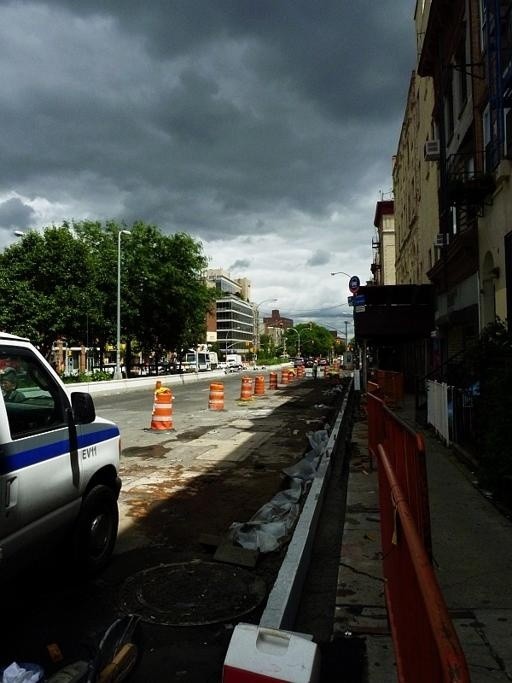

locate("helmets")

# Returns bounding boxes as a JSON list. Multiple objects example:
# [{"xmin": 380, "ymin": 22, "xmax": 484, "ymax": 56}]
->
[{"xmin": 0, "ymin": 367, "xmax": 18, "ymax": 384}]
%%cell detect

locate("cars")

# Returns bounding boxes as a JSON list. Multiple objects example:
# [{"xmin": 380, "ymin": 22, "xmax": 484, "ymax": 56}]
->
[
  {"xmin": 0, "ymin": 333, "xmax": 121, "ymax": 573},
  {"xmin": 294, "ymin": 358, "xmax": 329, "ymax": 368},
  {"xmin": 225, "ymin": 364, "xmax": 246, "ymax": 373},
  {"xmin": 218, "ymin": 362, "xmax": 228, "ymax": 369}
]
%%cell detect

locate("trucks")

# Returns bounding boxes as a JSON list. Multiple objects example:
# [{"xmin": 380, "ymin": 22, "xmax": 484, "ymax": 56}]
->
[{"xmin": 226, "ymin": 354, "xmax": 241, "ymax": 362}]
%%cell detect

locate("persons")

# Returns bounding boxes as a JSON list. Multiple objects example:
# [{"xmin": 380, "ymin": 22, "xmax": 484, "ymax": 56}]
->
[{"xmin": 0, "ymin": 367, "xmax": 25, "ymax": 403}]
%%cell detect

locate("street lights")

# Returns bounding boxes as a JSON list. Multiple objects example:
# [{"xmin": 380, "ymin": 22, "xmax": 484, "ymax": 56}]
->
[
  {"xmin": 286, "ymin": 328, "xmax": 311, "ymax": 359},
  {"xmin": 331, "ymin": 272, "xmax": 351, "ymax": 279},
  {"xmin": 116, "ymin": 230, "xmax": 131, "ymax": 380}
]
